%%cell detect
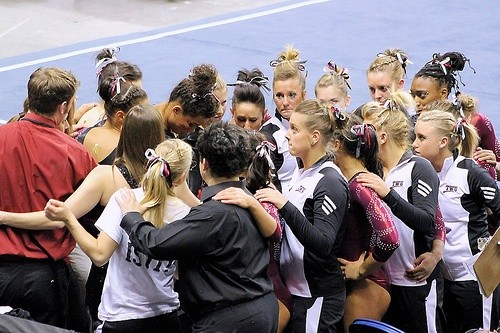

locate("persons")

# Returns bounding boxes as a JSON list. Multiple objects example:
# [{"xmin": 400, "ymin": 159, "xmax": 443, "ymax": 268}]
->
[
  {"xmin": 412, "ymin": 94, "xmax": 500, "ymax": 333},
  {"xmin": 0, "ymin": 104, "xmax": 167, "ymax": 324},
  {"xmin": 355, "ymin": 91, "xmax": 445, "ymax": 333},
  {"xmin": 211, "ymin": 130, "xmax": 293, "ymax": 333},
  {"xmin": 328, "ymin": 113, "xmax": 400, "ymax": 333},
  {"xmin": 253, "ymin": 98, "xmax": 351, "ymax": 333},
  {"xmin": 115, "ymin": 121, "xmax": 279, "ymax": 333},
  {"xmin": 6, "ymin": 46, "xmax": 149, "ymax": 238},
  {"xmin": 153, "ymin": 40, "xmax": 500, "ymax": 287},
  {"xmin": 44, "ymin": 139, "xmax": 193, "ymax": 333},
  {"xmin": 0, "ymin": 67, "xmax": 99, "ymax": 333}
]
